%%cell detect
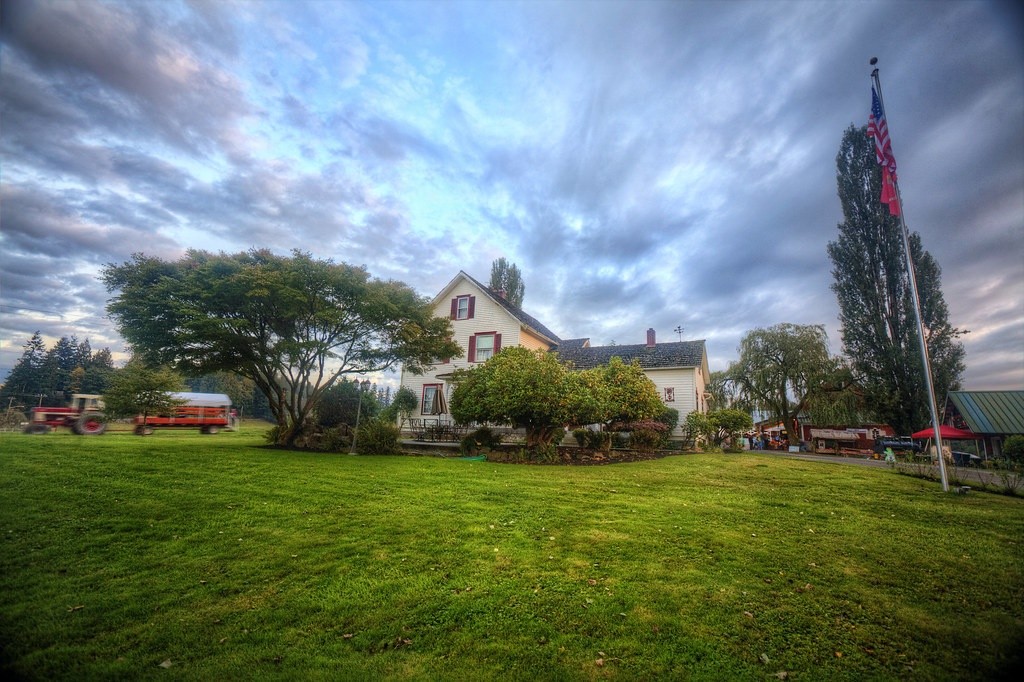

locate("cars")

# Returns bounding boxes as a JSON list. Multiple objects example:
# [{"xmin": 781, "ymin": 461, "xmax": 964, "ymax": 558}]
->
[{"xmin": 875, "ymin": 436, "xmax": 919, "ymax": 454}]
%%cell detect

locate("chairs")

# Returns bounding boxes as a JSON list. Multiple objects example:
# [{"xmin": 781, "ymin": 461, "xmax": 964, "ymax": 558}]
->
[{"xmin": 409, "ymin": 418, "xmax": 470, "ymax": 443}]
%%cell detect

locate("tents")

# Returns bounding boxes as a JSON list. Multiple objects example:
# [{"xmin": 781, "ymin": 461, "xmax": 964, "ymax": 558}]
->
[
  {"xmin": 911, "ymin": 425, "xmax": 982, "ymax": 466},
  {"xmin": 765, "ymin": 426, "xmax": 786, "ymax": 438}
]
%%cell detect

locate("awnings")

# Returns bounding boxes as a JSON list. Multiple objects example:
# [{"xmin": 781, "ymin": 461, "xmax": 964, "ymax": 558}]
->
[{"xmin": 810, "ymin": 428, "xmax": 861, "ymax": 440}]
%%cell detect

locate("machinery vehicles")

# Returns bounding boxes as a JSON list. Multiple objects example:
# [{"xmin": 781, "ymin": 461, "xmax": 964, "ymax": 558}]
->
[{"xmin": 29, "ymin": 391, "xmax": 237, "ymax": 435}]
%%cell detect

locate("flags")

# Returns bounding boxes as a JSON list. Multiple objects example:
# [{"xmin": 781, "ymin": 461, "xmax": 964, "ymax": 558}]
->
[{"xmin": 866, "ymin": 86, "xmax": 901, "ymax": 217}]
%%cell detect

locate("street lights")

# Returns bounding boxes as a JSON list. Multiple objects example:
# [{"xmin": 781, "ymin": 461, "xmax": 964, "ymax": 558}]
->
[{"xmin": 348, "ymin": 379, "xmax": 371, "ymax": 456}]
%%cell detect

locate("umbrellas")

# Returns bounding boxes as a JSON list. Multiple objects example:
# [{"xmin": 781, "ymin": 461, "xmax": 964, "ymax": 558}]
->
[{"xmin": 430, "ymin": 383, "xmax": 448, "ymax": 426}]
